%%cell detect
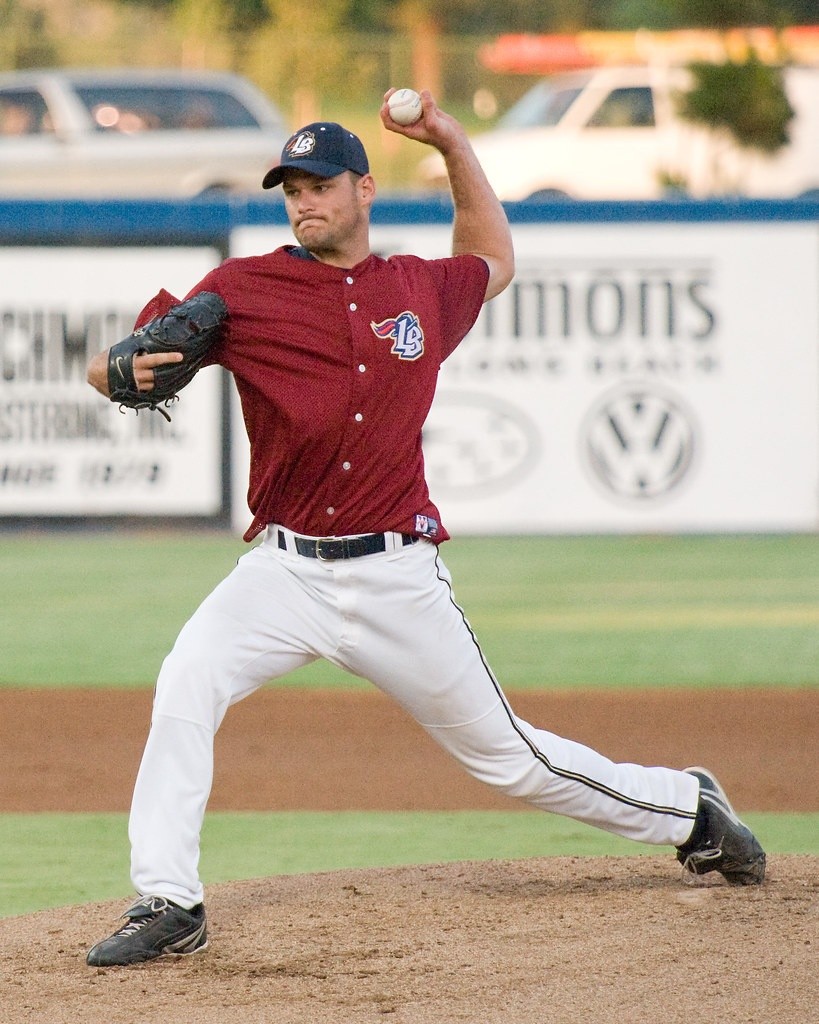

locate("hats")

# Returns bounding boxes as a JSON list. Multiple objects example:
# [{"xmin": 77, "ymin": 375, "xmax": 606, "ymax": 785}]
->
[{"xmin": 261, "ymin": 119, "xmax": 369, "ymax": 192}]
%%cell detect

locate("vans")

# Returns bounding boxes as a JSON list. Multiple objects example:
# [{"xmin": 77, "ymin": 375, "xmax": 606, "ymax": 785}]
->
[{"xmin": 429, "ymin": 60, "xmax": 819, "ymax": 201}]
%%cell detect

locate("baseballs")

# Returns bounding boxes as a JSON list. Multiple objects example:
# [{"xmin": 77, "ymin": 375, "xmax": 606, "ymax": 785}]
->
[{"xmin": 388, "ymin": 89, "xmax": 421, "ymax": 124}]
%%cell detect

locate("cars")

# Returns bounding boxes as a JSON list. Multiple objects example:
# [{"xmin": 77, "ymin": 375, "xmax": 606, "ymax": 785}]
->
[{"xmin": 0, "ymin": 69, "xmax": 289, "ymax": 203}]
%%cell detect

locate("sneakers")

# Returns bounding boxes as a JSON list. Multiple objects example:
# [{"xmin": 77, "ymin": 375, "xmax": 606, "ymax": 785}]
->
[
  {"xmin": 86, "ymin": 896, "xmax": 210, "ymax": 967},
  {"xmin": 676, "ymin": 764, "xmax": 769, "ymax": 887}
]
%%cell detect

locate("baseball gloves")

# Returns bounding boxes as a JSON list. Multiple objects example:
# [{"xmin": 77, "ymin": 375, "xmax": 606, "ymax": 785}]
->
[{"xmin": 107, "ymin": 289, "xmax": 226, "ymax": 421}]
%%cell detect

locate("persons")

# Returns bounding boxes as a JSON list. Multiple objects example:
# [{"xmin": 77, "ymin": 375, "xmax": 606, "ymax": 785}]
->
[{"xmin": 84, "ymin": 89, "xmax": 766, "ymax": 966}]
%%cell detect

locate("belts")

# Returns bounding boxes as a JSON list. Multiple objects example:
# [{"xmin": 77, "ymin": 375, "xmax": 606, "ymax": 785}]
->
[{"xmin": 278, "ymin": 532, "xmax": 419, "ymax": 562}]
